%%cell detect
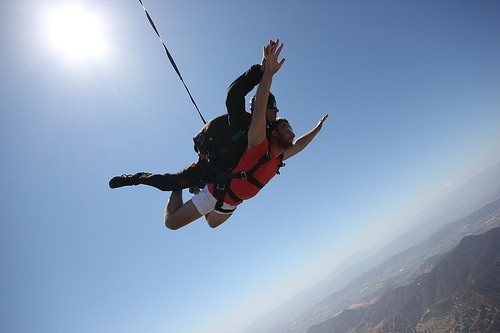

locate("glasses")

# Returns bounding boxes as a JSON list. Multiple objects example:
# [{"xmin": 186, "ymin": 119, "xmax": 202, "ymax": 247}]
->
[{"xmin": 267, "ymin": 98, "xmax": 276, "ymax": 110}]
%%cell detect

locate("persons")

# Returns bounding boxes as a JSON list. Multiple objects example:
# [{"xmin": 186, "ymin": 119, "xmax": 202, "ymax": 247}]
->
[
  {"xmin": 109, "ymin": 42, "xmax": 279, "ymax": 195},
  {"xmin": 164, "ymin": 39, "xmax": 329, "ymax": 230}
]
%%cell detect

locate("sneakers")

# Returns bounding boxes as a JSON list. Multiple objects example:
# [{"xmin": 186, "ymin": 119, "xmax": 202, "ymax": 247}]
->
[{"xmin": 109, "ymin": 174, "xmax": 134, "ymax": 188}]
